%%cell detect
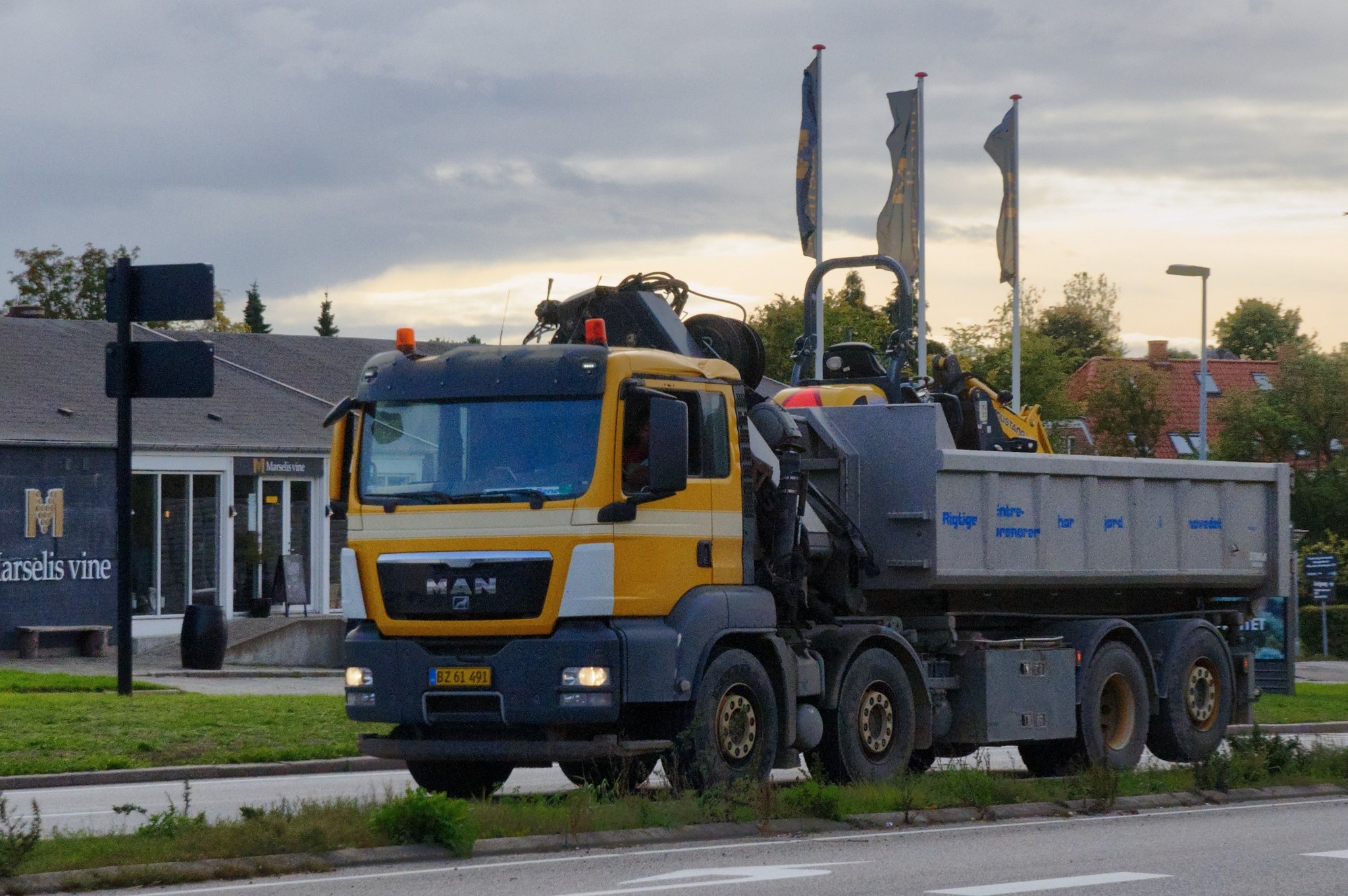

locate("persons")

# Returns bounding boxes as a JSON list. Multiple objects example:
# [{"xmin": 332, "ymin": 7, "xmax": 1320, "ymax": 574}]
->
[
  {"xmin": 619, "ymin": 407, "xmax": 651, "ymax": 494},
  {"xmin": 1246, "ymin": 595, "xmax": 1284, "ymax": 657}
]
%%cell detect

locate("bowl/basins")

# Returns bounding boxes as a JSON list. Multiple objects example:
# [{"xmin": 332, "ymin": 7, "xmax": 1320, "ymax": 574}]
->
[{"xmin": 249, "ymin": 597, "xmax": 272, "ymax": 618}]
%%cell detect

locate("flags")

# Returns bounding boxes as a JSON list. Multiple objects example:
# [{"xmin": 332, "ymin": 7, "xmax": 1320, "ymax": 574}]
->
[
  {"xmin": 875, "ymin": 87, "xmax": 924, "ymax": 279},
  {"xmin": 795, "ymin": 56, "xmax": 823, "ymax": 257},
  {"xmin": 983, "ymin": 107, "xmax": 1013, "ymax": 288}
]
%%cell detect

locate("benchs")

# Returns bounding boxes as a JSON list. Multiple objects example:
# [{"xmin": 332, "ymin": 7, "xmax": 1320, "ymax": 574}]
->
[{"xmin": 15, "ymin": 625, "xmax": 112, "ymax": 661}]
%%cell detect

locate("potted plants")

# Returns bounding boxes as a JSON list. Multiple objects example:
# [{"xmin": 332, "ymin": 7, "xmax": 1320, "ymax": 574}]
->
[{"xmin": 234, "ymin": 531, "xmax": 283, "ymax": 619}]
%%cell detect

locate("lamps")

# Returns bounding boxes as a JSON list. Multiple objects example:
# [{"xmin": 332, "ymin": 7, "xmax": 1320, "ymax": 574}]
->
[
  {"xmin": 325, "ymin": 504, "xmax": 334, "ymax": 518},
  {"xmin": 229, "ymin": 505, "xmax": 238, "ymax": 519}
]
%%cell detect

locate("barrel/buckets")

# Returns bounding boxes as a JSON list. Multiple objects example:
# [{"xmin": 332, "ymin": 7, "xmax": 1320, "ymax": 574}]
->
[{"xmin": 180, "ymin": 603, "xmax": 230, "ymax": 670}]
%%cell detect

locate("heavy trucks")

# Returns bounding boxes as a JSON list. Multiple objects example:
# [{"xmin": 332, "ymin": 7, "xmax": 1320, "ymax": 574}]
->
[{"xmin": 310, "ymin": 252, "xmax": 1300, "ymax": 807}]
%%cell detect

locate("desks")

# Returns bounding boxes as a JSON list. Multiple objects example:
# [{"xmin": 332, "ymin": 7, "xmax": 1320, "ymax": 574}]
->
[{"xmin": 194, "ymin": 588, "xmax": 237, "ymax": 595}]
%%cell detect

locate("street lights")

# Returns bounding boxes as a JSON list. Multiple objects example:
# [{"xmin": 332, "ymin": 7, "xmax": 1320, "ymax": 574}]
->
[{"xmin": 1165, "ymin": 262, "xmax": 1211, "ymax": 459}]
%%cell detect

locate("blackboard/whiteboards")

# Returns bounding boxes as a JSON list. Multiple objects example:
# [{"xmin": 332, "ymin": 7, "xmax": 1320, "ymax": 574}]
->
[{"xmin": 270, "ymin": 554, "xmax": 308, "ymax": 605}]
